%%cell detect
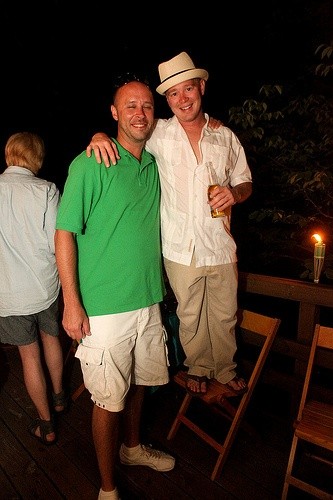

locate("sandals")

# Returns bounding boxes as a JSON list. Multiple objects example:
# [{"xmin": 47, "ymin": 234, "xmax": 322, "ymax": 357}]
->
[
  {"xmin": 27, "ymin": 418, "xmax": 56, "ymax": 445},
  {"xmin": 52, "ymin": 390, "xmax": 65, "ymax": 414}
]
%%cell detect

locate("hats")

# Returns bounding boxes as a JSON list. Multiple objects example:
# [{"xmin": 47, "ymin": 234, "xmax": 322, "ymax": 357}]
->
[{"xmin": 156, "ymin": 52, "xmax": 209, "ymax": 95}]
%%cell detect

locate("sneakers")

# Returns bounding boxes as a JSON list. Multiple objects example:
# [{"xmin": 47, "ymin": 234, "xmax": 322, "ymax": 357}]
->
[{"xmin": 120, "ymin": 444, "xmax": 175, "ymax": 471}]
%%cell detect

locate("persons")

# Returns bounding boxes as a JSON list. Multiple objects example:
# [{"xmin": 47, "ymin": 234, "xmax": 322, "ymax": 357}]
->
[
  {"xmin": 87, "ymin": 51, "xmax": 252, "ymax": 397},
  {"xmin": 54, "ymin": 81, "xmax": 175, "ymax": 500},
  {"xmin": 0, "ymin": 135, "xmax": 65, "ymax": 441}
]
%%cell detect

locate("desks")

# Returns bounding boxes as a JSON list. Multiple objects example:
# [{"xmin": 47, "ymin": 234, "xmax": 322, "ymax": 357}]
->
[{"xmin": 238, "ymin": 272, "xmax": 333, "ymax": 381}]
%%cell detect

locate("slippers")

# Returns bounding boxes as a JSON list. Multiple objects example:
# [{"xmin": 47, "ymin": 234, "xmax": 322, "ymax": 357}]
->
[
  {"xmin": 186, "ymin": 375, "xmax": 209, "ymax": 398},
  {"xmin": 224, "ymin": 374, "xmax": 248, "ymax": 395}
]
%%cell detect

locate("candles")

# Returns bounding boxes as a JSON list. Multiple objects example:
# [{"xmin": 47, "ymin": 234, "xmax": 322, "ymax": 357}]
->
[{"xmin": 308, "ymin": 230, "xmax": 330, "ymax": 285}]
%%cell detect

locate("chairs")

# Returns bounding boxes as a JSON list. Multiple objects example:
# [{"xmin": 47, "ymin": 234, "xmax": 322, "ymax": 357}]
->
[
  {"xmin": 166, "ymin": 309, "xmax": 280, "ymax": 481},
  {"xmin": 280, "ymin": 323, "xmax": 333, "ymax": 498}
]
